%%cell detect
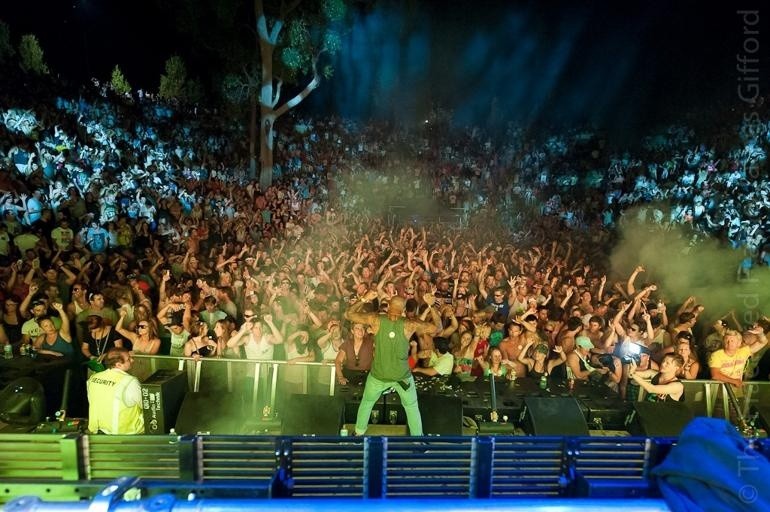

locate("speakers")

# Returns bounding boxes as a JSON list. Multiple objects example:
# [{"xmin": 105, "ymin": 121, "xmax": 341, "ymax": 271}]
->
[
  {"xmin": 621, "ymin": 401, "xmax": 695, "ymax": 437},
  {"xmin": 407, "ymin": 391, "xmax": 463, "ymax": 442},
  {"xmin": 517, "ymin": 398, "xmax": 590, "ymax": 437},
  {"xmin": 281, "ymin": 394, "xmax": 345, "ymax": 436},
  {"xmin": 175, "ymin": 391, "xmax": 242, "ymax": 435}
]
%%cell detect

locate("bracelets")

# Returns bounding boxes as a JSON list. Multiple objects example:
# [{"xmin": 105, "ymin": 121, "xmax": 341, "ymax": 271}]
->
[
  {"xmin": 429, "ymin": 304, "xmax": 435, "ymax": 309},
  {"xmin": 359, "ymin": 296, "xmax": 367, "ymax": 304}
]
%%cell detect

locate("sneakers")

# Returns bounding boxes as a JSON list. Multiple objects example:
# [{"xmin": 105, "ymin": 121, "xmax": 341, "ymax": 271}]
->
[{"xmin": 351, "ymin": 431, "xmax": 362, "ymax": 445}]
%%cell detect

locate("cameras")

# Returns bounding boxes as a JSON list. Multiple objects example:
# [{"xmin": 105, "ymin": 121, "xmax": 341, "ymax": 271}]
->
[{"xmin": 621, "ymin": 352, "xmax": 641, "ymax": 366}]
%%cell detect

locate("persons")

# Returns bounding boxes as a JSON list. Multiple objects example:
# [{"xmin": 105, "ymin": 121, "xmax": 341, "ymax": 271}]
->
[
  {"xmin": 86, "ymin": 350, "xmax": 146, "ymax": 436},
  {"xmin": 709, "ymin": 327, "xmax": 768, "ymax": 416},
  {"xmin": 347, "ymin": 288, "xmax": 443, "ymax": 454},
  {"xmin": 0, "ymin": 74, "xmax": 770, "ymax": 381},
  {"xmin": 629, "ymin": 353, "xmax": 684, "ymax": 403}
]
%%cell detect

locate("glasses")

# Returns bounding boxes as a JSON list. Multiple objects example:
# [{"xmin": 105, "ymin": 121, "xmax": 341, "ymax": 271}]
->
[
  {"xmin": 627, "ymin": 325, "xmax": 637, "ymax": 332},
  {"xmin": 135, "ymin": 324, "xmax": 150, "ymax": 329},
  {"xmin": 243, "ymin": 314, "xmax": 255, "ymax": 318},
  {"xmin": 73, "ymin": 287, "xmax": 84, "ymax": 291}
]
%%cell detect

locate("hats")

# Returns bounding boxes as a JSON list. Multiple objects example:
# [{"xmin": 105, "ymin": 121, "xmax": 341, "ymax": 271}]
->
[
  {"xmin": 327, "ymin": 320, "xmax": 341, "ymax": 333},
  {"xmin": 534, "ymin": 344, "xmax": 549, "ymax": 355},
  {"xmin": 575, "ymin": 336, "xmax": 595, "ymax": 349}
]
